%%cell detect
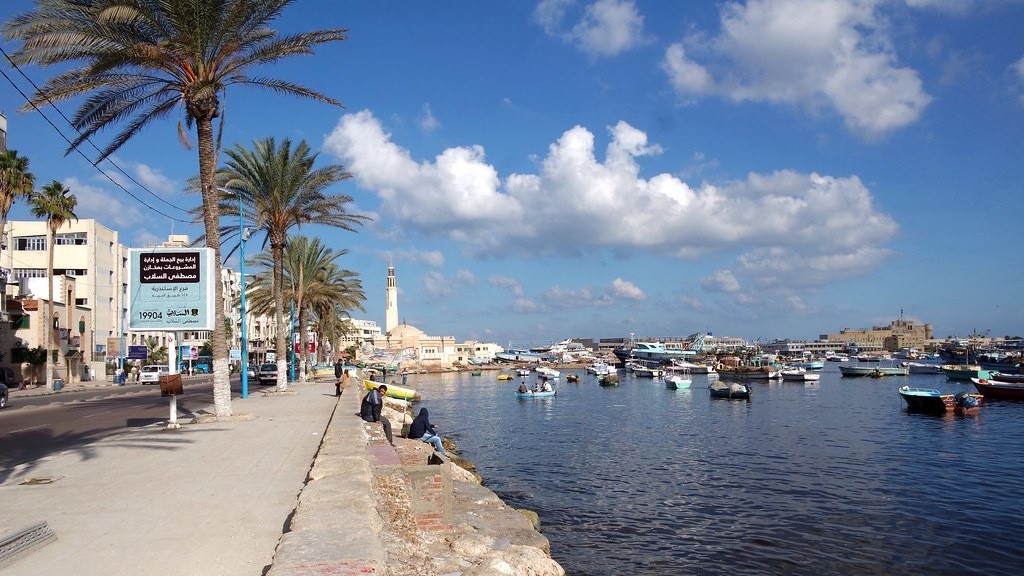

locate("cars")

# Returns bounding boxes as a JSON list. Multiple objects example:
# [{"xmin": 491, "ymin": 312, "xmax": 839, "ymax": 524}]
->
[
  {"xmin": 0, "ymin": 384, "xmax": 10, "ymax": 406},
  {"xmin": 240, "ymin": 367, "xmax": 259, "ymax": 381}
]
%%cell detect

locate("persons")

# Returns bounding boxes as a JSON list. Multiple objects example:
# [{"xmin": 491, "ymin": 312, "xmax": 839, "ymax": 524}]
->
[
  {"xmin": 361, "ymin": 385, "xmax": 392, "ymax": 443},
  {"xmin": 84, "ymin": 365, "xmax": 89, "ymax": 381},
  {"xmin": 408, "ymin": 407, "xmax": 447, "ymax": 456},
  {"xmin": 370, "ymin": 372, "xmax": 375, "ymax": 381},
  {"xmin": 383, "ymin": 367, "xmax": 387, "ymax": 381},
  {"xmin": 401, "ymin": 368, "xmax": 408, "ymax": 384},
  {"xmin": 532, "ymin": 382, "xmax": 540, "ymax": 392},
  {"xmin": 335, "ymin": 359, "xmax": 343, "ymax": 396},
  {"xmin": 131, "ymin": 366, "xmax": 140, "ymax": 382},
  {"xmin": 338, "ymin": 369, "xmax": 351, "ymax": 391},
  {"xmin": 518, "ymin": 382, "xmax": 527, "ymax": 393},
  {"xmin": 543, "ymin": 381, "xmax": 552, "ymax": 391},
  {"xmin": 119, "ymin": 370, "xmax": 126, "ymax": 381}
]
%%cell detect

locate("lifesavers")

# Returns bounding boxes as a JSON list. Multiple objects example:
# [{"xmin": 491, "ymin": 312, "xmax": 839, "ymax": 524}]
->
[{"xmin": 658, "ymin": 371, "xmax": 663, "ymax": 376}]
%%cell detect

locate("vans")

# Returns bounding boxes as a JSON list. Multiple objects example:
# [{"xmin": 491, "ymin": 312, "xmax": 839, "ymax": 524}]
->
[{"xmin": 180, "ymin": 356, "xmax": 213, "ymax": 374}]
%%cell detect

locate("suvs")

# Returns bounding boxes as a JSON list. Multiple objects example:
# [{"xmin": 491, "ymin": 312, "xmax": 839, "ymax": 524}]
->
[
  {"xmin": 258, "ymin": 363, "xmax": 278, "ymax": 385},
  {"xmin": 140, "ymin": 365, "xmax": 170, "ymax": 384}
]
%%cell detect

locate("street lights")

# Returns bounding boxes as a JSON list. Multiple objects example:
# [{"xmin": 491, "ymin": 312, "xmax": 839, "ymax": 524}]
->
[{"xmin": 217, "ymin": 187, "xmax": 251, "ymax": 398}]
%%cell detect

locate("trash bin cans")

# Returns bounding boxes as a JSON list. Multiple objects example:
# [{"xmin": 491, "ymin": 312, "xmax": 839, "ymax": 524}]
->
[{"xmin": 54, "ymin": 380, "xmax": 61, "ymax": 390}]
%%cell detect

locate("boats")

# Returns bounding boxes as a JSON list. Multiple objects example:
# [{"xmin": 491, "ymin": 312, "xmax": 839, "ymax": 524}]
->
[
  {"xmin": 727, "ymin": 381, "xmax": 754, "ymax": 401},
  {"xmin": 900, "ymin": 387, "xmax": 958, "ymax": 414},
  {"xmin": 535, "ymin": 366, "xmax": 562, "ymax": 381},
  {"xmin": 627, "ymin": 360, "xmax": 693, "ymax": 389},
  {"xmin": 970, "ymin": 376, "xmax": 1024, "ymax": 400},
  {"xmin": 989, "ymin": 370, "xmax": 1024, "ymax": 384},
  {"xmin": 953, "ymin": 392, "xmax": 985, "ymax": 414},
  {"xmin": 518, "ymin": 367, "xmax": 531, "ymax": 377},
  {"xmin": 707, "ymin": 379, "xmax": 731, "ymax": 398},
  {"xmin": 472, "ymin": 370, "xmax": 482, "ymax": 375},
  {"xmin": 857, "ymin": 356, "xmax": 882, "ymax": 362},
  {"xmin": 514, "ymin": 389, "xmax": 562, "ymax": 399},
  {"xmin": 942, "ymin": 349, "xmax": 987, "ymax": 380},
  {"xmin": 900, "ymin": 361, "xmax": 948, "ymax": 373},
  {"xmin": 566, "ymin": 373, "xmax": 581, "ymax": 382},
  {"xmin": 583, "ymin": 360, "xmax": 621, "ymax": 388},
  {"xmin": 837, "ymin": 357, "xmax": 911, "ymax": 378},
  {"xmin": 363, "ymin": 379, "xmax": 422, "ymax": 403},
  {"xmin": 663, "ymin": 351, "xmax": 849, "ymax": 383},
  {"xmin": 498, "ymin": 373, "xmax": 515, "ymax": 380}
]
